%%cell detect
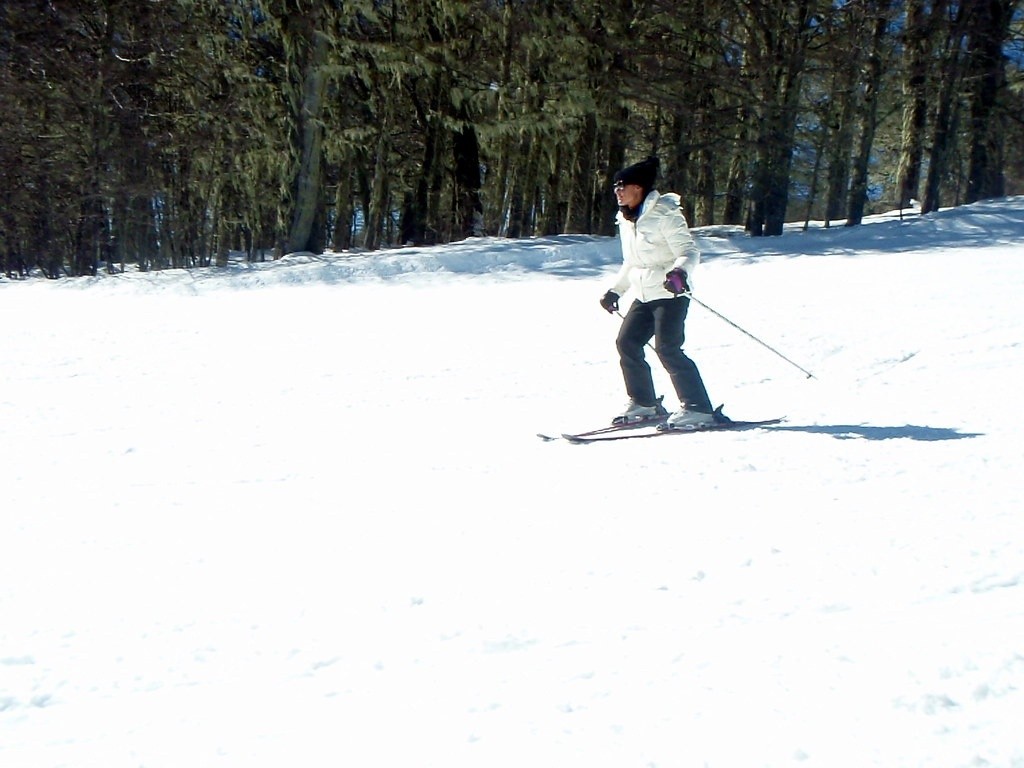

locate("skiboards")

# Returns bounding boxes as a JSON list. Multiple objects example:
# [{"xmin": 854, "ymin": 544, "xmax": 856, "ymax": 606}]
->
[{"xmin": 535, "ymin": 403, "xmax": 780, "ymax": 444}]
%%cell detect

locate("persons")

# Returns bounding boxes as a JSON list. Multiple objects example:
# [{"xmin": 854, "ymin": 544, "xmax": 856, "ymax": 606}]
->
[{"xmin": 600, "ymin": 156, "xmax": 715, "ymax": 425}]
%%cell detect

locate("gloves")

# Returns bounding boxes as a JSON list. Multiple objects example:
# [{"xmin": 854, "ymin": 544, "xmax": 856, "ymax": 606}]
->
[
  {"xmin": 663, "ymin": 268, "xmax": 687, "ymax": 294},
  {"xmin": 600, "ymin": 292, "xmax": 620, "ymax": 314}
]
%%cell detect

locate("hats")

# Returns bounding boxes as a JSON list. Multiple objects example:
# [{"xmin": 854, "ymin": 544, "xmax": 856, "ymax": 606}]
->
[{"xmin": 614, "ymin": 156, "xmax": 659, "ymax": 189}]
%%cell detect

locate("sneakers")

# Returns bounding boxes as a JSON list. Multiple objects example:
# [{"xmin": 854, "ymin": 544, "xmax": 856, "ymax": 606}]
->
[
  {"xmin": 612, "ymin": 395, "xmax": 667, "ymax": 426},
  {"xmin": 656, "ymin": 407, "xmax": 716, "ymax": 430}
]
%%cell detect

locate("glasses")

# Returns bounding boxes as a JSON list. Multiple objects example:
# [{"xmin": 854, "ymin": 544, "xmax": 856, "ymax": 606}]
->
[{"xmin": 613, "ymin": 180, "xmax": 633, "ymax": 190}]
